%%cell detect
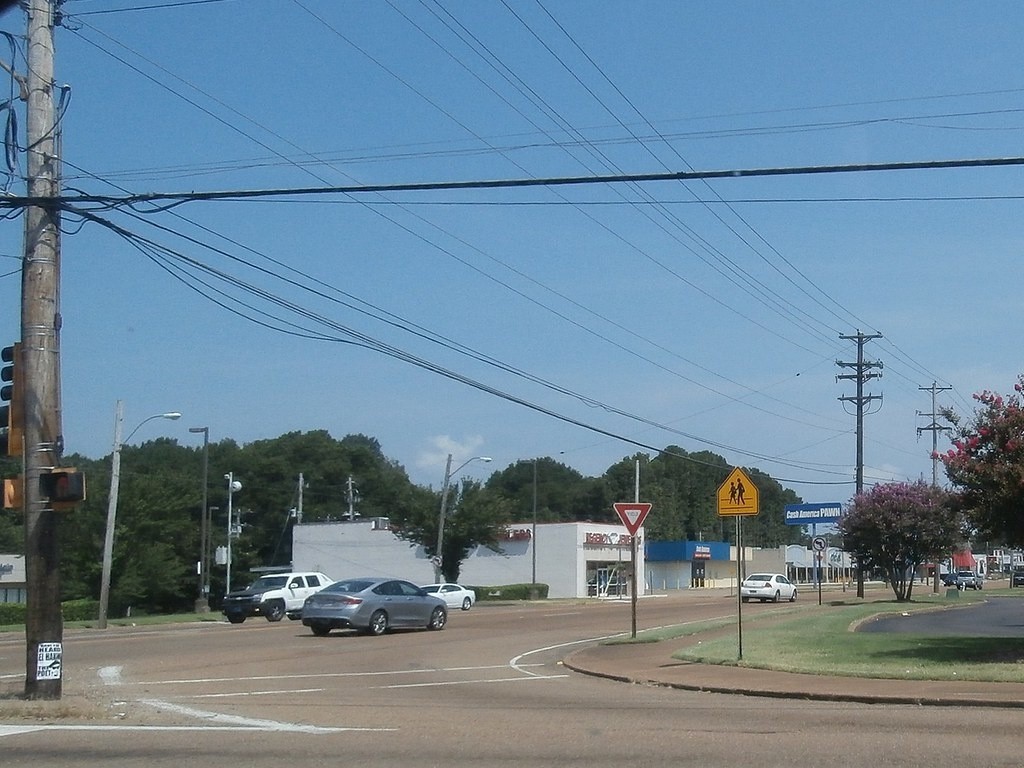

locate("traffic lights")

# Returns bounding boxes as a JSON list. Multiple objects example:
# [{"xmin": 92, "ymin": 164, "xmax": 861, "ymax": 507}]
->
[{"xmin": 40, "ymin": 471, "xmax": 82, "ymax": 504}]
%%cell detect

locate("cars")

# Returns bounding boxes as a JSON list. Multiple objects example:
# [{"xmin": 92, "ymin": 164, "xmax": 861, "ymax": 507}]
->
[
  {"xmin": 741, "ymin": 572, "xmax": 798, "ymax": 603},
  {"xmin": 1013, "ymin": 573, "xmax": 1024, "ymax": 587},
  {"xmin": 302, "ymin": 577, "xmax": 448, "ymax": 636},
  {"xmin": 418, "ymin": 583, "xmax": 476, "ymax": 611},
  {"xmin": 943, "ymin": 574, "xmax": 956, "ymax": 587}
]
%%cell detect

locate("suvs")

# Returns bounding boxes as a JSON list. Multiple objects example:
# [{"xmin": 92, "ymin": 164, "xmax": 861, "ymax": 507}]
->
[
  {"xmin": 221, "ymin": 571, "xmax": 335, "ymax": 623},
  {"xmin": 956, "ymin": 570, "xmax": 983, "ymax": 590}
]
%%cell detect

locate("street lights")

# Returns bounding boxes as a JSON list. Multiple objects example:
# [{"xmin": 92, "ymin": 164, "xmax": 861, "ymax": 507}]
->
[
  {"xmin": 95, "ymin": 398, "xmax": 183, "ymax": 631},
  {"xmin": 432, "ymin": 454, "xmax": 493, "ymax": 583}
]
